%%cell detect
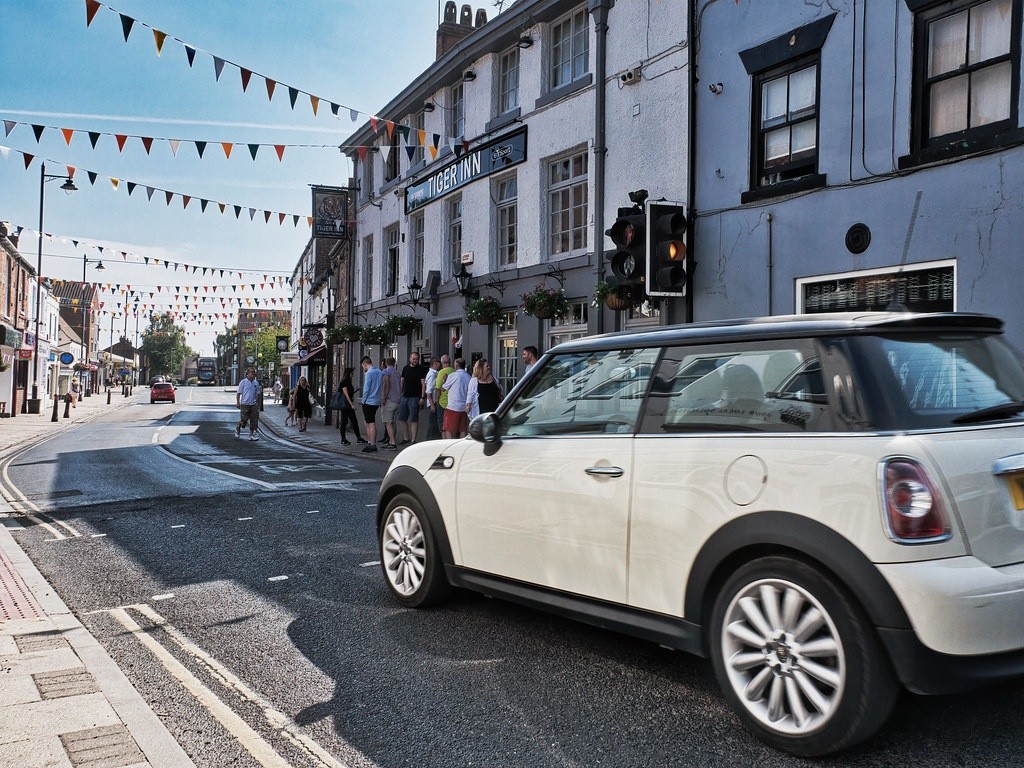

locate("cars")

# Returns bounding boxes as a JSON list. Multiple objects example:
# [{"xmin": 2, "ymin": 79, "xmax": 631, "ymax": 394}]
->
[
  {"xmin": 149, "ymin": 377, "xmax": 163, "ymax": 388},
  {"xmin": 150, "ymin": 382, "xmax": 177, "ymax": 403}
]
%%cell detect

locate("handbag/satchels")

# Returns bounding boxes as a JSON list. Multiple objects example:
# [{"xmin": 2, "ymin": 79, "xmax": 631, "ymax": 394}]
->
[
  {"xmin": 329, "ymin": 390, "xmax": 349, "ymax": 409},
  {"xmin": 307, "ymin": 386, "xmax": 314, "ymax": 404},
  {"xmin": 64, "ymin": 393, "xmax": 72, "ymax": 402}
]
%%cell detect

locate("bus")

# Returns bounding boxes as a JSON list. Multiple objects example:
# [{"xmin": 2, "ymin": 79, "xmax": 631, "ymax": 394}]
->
[{"xmin": 197, "ymin": 358, "xmax": 217, "ymax": 386}]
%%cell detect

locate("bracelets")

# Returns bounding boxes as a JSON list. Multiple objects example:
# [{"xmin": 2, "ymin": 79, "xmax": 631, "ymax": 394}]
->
[{"xmin": 421, "ymin": 397, "xmax": 425, "ymax": 400}]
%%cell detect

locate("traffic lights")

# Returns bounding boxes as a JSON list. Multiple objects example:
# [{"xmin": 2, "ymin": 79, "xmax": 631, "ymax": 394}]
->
[
  {"xmin": 605, "ymin": 207, "xmax": 646, "ymax": 310},
  {"xmin": 646, "ymin": 200, "xmax": 687, "ymax": 297}
]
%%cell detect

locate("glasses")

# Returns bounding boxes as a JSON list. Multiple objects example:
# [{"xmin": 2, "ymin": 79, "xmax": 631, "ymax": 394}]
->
[{"xmin": 300, "ymin": 380, "xmax": 305, "ymax": 381}]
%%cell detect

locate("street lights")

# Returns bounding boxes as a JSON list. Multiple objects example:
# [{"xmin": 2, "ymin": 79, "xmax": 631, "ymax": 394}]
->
[
  {"xmin": 26, "ymin": 161, "xmax": 79, "ymax": 414},
  {"xmin": 171, "ymin": 347, "xmax": 175, "ymax": 382},
  {"xmin": 122, "ymin": 292, "xmax": 140, "ymax": 395},
  {"xmin": 77, "ymin": 254, "xmax": 106, "ymax": 401},
  {"xmin": 111, "ymin": 316, "xmax": 120, "ymax": 385}
]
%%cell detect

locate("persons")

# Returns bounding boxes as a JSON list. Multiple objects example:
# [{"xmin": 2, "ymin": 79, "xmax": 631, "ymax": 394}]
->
[
  {"xmin": 273, "ymin": 381, "xmax": 282, "ymax": 403},
  {"xmin": 285, "ymin": 377, "xmax": 311, "ymax": 432},
  {"xmin": 522, "ymin": 346, "xmax": 551, "ymax": 421},
  {"xmin": 235, "ymin": 367, "xmax": 259, "ymax": 441},
  {"xmin": 465, "ymin": 359, "xmax": 505, "ymax": 422},
  {"xmin": 360, "ymin": 356, "xmax": 382, "ymax": 454},
  {"xmin": 397, "ymin": 352, "xmax": 426, "ymax": 446},
  {"xmin": 425, "ymin": 355, "xmax": 456, "ymax": 440},
  {"xmin": 115, "ymin": 374, "xmax": 123, "ymax": 386},
  {"xmin": 161, "ymin": 375, "xmax": 171, "ymax": 382},
  {"xmin": 338, "ymin": 368, "xmax": 368, "ymax": 446},
  {"xmin": 441, "ymin": 358, "xmax": 472, "ymax": 439},
  {"xmin": 377, "ymin": 358, "xmax": 401, "ymax": 451},
  {"xmin": 70, "ymin": 377, "xmax": 79, "ymax": 408}
]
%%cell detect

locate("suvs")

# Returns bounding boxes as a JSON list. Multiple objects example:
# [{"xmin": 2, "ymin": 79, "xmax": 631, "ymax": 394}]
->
[{"xmin": 376, "ymin": 312, "xmax": 1024, "ymax": 761}]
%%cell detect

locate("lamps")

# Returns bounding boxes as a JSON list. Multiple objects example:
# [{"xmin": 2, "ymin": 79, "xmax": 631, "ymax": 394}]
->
[
  {"xmin": 407, "ymin": 276, "xmax": 430, "ymax": 312},
  {"xmin": 454, "ymin": 263, "xmax": 479, "ymax": 301},
  {"xmin": 462, "ymin": 71, "xmax": 476, "ymax": 82},
  {"xmin": 516, "ymin": 36, "xmax": 532, "ymax": 48},
  {"xmin": 370, "ymin": 147, "xmax": 378, "ymax": 152},
  {"xmin": 394, "ymin": 126, "xmax": 404, "ymax": 133},
  {"xmin": 423, "ymin": 103, "xmax": 434, "ymax": 112}
]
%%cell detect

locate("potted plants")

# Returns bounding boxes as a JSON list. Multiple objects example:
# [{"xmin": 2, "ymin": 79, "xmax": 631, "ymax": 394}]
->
[{"xmin": 279, "ymin": 387, "xmax": 289, "ymax": 406}]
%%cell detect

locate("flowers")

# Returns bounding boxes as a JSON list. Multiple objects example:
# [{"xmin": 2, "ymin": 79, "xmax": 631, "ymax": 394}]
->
[
  {"xmin": 464, "ymin": 296, "xmax": 506, "ymax": 327},
  {"xmin": 324, "ymin": 313, "xmax": 420, "ymax": 348},
  {"xmin": 519, "ymin": 280, "xmax": 570, "ymax": 319},
  {"xmin": 590, "ymin": 280, "xmax": 646, "ymax": 311}
]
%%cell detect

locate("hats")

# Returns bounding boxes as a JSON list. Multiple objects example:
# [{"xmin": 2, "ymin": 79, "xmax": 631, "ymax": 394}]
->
[{"xmin": 72, "ymin": 377, "xmax": 77, "ymax": 381}]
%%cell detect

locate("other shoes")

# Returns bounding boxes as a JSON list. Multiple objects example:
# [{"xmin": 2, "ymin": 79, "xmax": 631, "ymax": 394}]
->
[
  {"xmin": 285, "ymin": 420, "xmax": 288, "ymax": 426},
  {"xmin": 361, "ymin": 445, "xmax": 377, "ymax": 454},
  {"xmin": 398, "ymin": 439, "xmax": 410, "ymax": 445},
  {"xmin": 379, "ymin": 443, "xmax": 397, "ymax": 450},
  {"xmin": 341, "ymin": 440, "xmax": 350, "ymax": 446},
  {"xmin": 303, "ymin": 424, "xmax": 306, "ymax": 431},
  {"xmin": 356, "ymin": 440, "xmax": 368, "ymax": 444},
  {"xmin": 235, "ymin": 426, "xmax": 240, "ymax": 438},
  {"xmin": 299, "ymin": 428, "xmax": 302, "ymax": 432},
  {"xmin": 248, "ymin": 435, "xmax": 259, "ymax": 441}
]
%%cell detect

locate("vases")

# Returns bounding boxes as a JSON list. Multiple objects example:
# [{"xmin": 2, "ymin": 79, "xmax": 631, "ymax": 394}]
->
[
  {"xmin": 392, "ymin": 327, "xmax": 409, "ymax": 336},
  {"xmin": 532, "ymin": 305, "xmax": 556, "ymax": 319},
  {"xmin": 331, "ymin": 337, "xmax": 345, "ymax": 345},
  {"xmin": 367, "ymin": 337, "xmax": 382, "ymax": 345},
  {"xmin": 475, "ymin": 314, "xmax": 497, "ymax": 325},
  {"xmin": 347, "ymin": 335, "xmax": 361, "ymax": 342},
  {"xmin": 604, "ymin": 292, "xmax": 632, "ymax": 310}
]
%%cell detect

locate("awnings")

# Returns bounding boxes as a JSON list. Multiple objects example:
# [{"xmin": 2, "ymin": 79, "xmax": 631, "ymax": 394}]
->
[{"xmin": 294, "ymin": 345, "xmax": 326, "ymax": 366}]
